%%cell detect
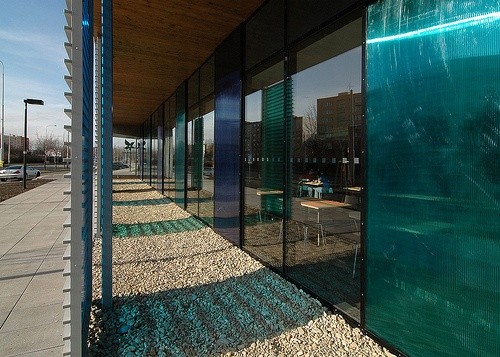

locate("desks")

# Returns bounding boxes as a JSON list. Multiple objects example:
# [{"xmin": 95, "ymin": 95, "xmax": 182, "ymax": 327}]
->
[{"xmin": 255, "ymin": 175, "xmax": 362, "ymax": 249}]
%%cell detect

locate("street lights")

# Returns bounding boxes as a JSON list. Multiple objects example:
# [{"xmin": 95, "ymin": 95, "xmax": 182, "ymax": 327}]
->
[{"xmin": 23, "ymin": 98, "xmax": 44, "ymax": 189}]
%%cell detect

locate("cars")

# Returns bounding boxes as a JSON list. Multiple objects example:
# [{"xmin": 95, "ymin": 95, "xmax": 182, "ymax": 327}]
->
[{"xmin": 0, "ymin": 164, "xmax": 41, "ymax": 182}]
